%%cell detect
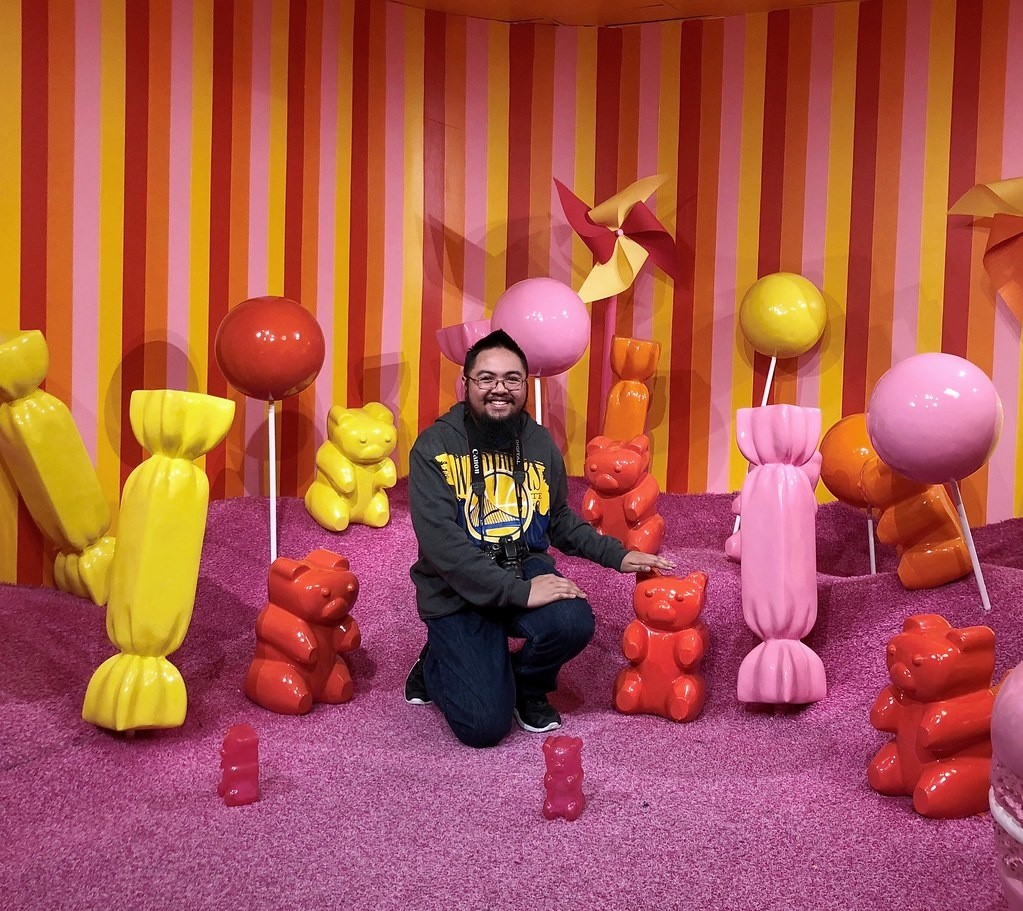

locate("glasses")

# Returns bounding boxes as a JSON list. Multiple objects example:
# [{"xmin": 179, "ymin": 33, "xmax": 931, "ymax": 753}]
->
[{"xmin": 468, "ymin": 375, "xmax": 526, "ymax": 390}]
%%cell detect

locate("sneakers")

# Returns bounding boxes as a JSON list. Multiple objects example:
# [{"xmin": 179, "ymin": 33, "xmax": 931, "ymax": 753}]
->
[
  {"xmin": 509, "ymin": 651, "xmax": 562, "ymax": 732},
  {"xmin": 403, "ymin": 641, "xmax": 433, "ymax": 704}
]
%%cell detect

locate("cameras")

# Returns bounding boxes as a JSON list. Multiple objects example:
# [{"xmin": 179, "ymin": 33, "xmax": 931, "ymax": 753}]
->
[{"xmin": 484, "ymin": 535, "xmax": 533, "ymax": 580}]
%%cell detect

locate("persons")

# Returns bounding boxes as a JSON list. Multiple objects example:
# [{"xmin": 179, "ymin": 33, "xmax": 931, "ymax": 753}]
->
[{"xmin": 404, "ymin": 328, "xmax": 678, "ymax": 749}]
